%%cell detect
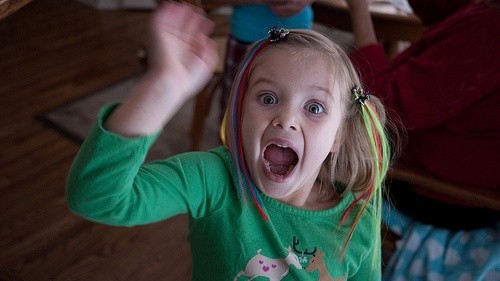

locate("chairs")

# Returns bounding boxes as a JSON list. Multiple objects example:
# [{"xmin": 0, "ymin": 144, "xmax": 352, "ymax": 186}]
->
[{"xmin": 382, "ymin": 161, "xmax": 500, "ymax": 253}]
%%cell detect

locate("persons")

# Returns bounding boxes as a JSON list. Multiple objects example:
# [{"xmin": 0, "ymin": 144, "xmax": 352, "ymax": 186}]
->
[
  {"xmin": 64, "ymin": 2, "xmax": 383, "ymax": 281},
  {"xmin": 344, "ymin": 0, "xmax": 500, "ymax": 229},
  {"xmin": 219, "ymin": 0, "xmax": 315, "ymax": 150}
]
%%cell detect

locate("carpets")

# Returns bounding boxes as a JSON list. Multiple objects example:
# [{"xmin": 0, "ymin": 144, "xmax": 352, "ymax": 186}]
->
[{"xmin": 33, "ymin": 67, "xmax": 220, "ymax": 161}]
